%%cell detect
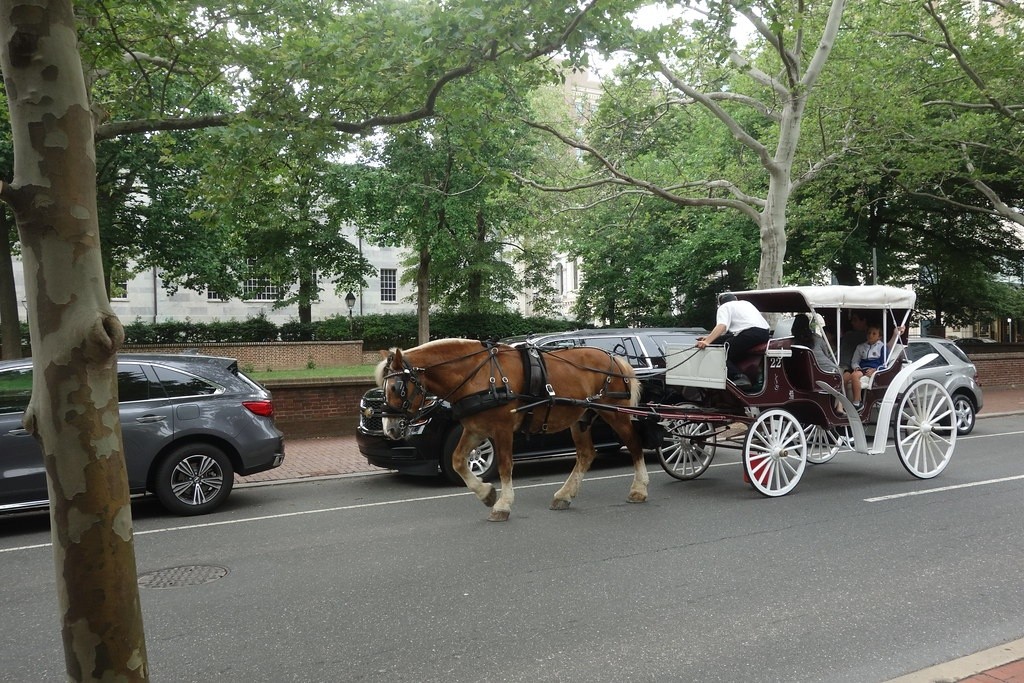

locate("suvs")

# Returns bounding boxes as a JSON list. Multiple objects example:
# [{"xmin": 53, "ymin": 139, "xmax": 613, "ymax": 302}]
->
[
  {"xmin": 1, "ymin": 351, "xmax": 286, "ymax": 518},
  {"xmin": 355, "ymin": 327, "xmax": 725, "ymax": 487},
  {"xmin": 817, "ymin": 333, "xmax": 983, "ymax": 449}
]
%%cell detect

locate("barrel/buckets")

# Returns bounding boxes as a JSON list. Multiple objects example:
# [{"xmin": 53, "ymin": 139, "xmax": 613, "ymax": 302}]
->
[{"xmin": 744, "ymin": 455, "xmax": 769, "ymax": 485}]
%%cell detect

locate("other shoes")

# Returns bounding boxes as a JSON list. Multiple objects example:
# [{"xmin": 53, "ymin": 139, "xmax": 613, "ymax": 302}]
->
[
  {"xmin": 732, "ymin": 378, "xmax": 751, "ymax": 390},
  {"xmin": 834, "ymin": 408, "xmax": 847, "ymax": 418}
]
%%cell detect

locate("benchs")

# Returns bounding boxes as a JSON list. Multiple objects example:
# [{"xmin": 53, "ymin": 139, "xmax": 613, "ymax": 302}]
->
[
  {"xmin": 697, "ymin": 335, "xmax": 797, "ymax": 359},
  {"xmin": 835, "ymin": 343, "xmax": 909, "ymax": 384},
  {"xmin": 789, "ymin": 344, "xmax": 840, "ymax": 391}
]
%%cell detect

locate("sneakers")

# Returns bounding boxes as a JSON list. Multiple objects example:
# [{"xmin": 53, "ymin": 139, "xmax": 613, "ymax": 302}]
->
[{"xmin": 852, "ymin": 400, "xmax": 864, "ymax": 413}]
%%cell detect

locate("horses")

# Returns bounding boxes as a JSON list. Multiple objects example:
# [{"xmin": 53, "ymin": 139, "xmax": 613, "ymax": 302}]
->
[{"xmin": 374, "ymin": 338, "xmax": 650, "ymax": 523}]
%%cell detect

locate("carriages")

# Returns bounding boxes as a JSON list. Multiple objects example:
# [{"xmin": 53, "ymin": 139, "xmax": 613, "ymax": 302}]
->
[{"xmin": 375, "ymin": 285, "xmax": 957, "ymax": 523}]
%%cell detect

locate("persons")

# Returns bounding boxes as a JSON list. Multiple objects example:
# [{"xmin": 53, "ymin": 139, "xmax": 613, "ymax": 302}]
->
[
  {"xmin": 790, "ymin": 308, "xmax": 906, "ymax": 413},
  {"xmin": 695, "ymin": 294, "xmax": 769, "ymax": 388}
]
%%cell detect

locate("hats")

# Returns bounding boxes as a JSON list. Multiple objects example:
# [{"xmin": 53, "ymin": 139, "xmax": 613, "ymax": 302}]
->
[{"xmin": 718, "ymin": 293, "xmax": 738, "ymax": 307}]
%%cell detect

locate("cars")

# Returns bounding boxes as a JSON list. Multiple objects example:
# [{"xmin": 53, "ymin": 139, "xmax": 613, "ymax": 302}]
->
[{"xmin": 949, "ymin": 336, "xmax": 996, "ymax": 355}]
